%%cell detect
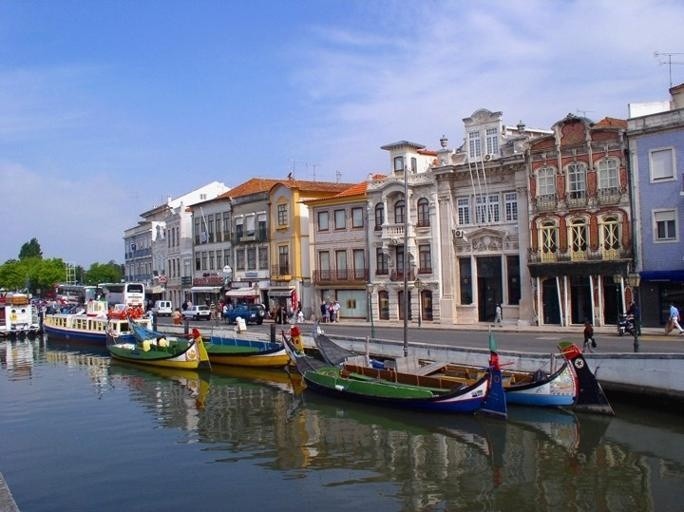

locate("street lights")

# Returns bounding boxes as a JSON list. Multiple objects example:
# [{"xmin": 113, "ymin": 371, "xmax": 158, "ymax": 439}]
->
[
  {"xmin": 628, "ymin": 273, "xmax": 641, "ymax": 353},
  {"xmin": 414, "ymin": 278, "xmax": 423, "ymax": 328},
  {"xmin": 366, "ymin": 284, "xmax": 375, "ymax": 339},
  {"xmin": 613, "ymin": 274, "xmax": 622, "ymax": 332}
]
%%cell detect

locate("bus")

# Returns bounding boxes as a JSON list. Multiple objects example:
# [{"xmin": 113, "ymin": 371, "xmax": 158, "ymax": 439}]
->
[{"xmin": 57, "ymin": 283, "xmax": 145, "ymax": 315}]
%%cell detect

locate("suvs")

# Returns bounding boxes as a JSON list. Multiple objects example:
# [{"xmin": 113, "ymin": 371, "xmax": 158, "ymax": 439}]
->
[{"xmin": 224, "ymin": 304, "xmax": 266, "ymax": 324}]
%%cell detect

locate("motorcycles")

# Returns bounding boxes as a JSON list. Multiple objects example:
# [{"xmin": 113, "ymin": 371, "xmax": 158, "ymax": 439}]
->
[{"xmin": 619, "ymin": 312, "xmax": 639, "ymax": 336}]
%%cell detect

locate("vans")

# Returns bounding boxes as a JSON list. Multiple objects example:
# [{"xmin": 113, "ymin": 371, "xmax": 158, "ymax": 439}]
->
[{"xmin": 152, "ymin": 300, "xmax": 172, "ymax": 316}]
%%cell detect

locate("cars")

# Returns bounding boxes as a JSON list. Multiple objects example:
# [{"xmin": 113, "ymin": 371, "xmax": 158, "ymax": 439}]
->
[{"xmin": 181, "ymin": 305, "xmax": 212, "ymax": 321}]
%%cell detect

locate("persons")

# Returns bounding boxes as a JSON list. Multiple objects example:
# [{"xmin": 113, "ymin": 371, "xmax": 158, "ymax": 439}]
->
[
  {"xmin": 665, "ymin": 303, "xmax": 683, "ymax": 336},
  {"xmin": 182, "ymin": 298, "xmax": 192, "ymax": 310},
  {"xmin": 260, "ymin": 301, "xmax": 271, "ymax": 320},
  {"xmin": 205, "ymin": 298, "xmax": 233, "ymax": 325},
  {"xmin": 627, "ymin": 301, "xmax": 642, "ymax": 336},
  {"xmin": 582, "ymin": 318, "xmax": 597, "ymax": 353},
  {"xmin": 171, "ymin": 308, "xmax": 184, "ymax": 325},
  {"xmin": 321, "ymin": 299, "xmax": 340, "ymax": 323},
  {"xmin": 296, "ymin": 301, "xmax": 304, "ymax": 323},
  {"xmin": 492, "ymin": 303, "xmax": 504, "ymax": 327},
  {"xmin": 107, "ymin": 302, "xmax": 144, "ymax": 320}
]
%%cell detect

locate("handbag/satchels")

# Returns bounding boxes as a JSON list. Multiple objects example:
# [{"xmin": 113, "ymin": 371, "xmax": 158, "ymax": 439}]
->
[
  {"xmin": 668, "ymin": 321, "xmax": 672, "ymax": 332},
  {"xmin": 592, "ymin": 339, "xmax": 597, "ymax": 347}
]
